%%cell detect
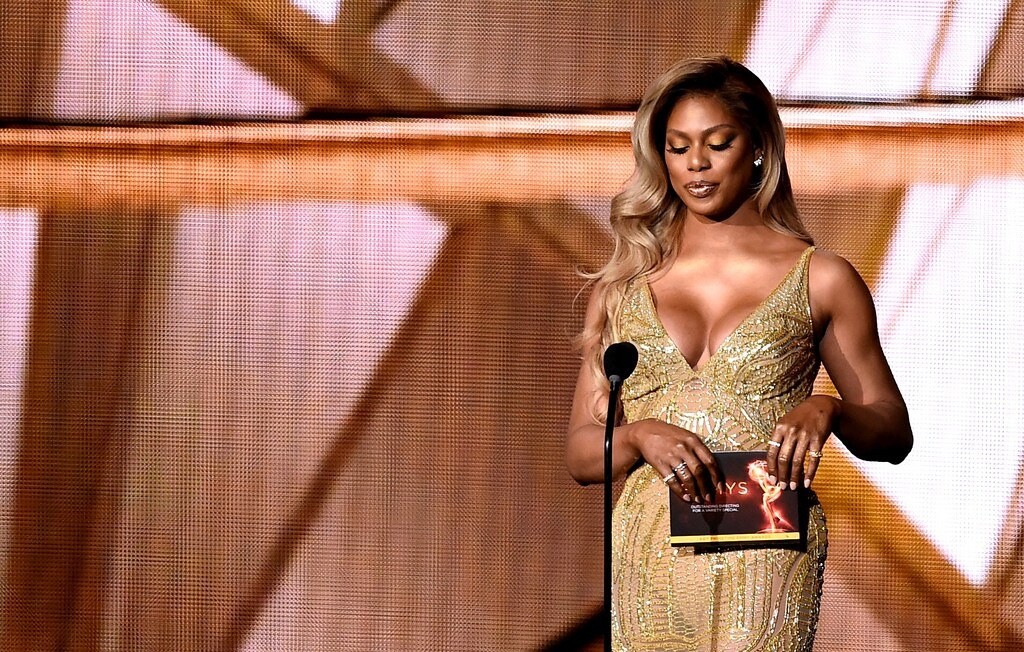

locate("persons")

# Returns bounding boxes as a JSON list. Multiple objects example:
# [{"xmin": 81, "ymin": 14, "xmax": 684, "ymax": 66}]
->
[{"xmin": 564, "ymin": 51, "xmax": 915, "ymax": 652}]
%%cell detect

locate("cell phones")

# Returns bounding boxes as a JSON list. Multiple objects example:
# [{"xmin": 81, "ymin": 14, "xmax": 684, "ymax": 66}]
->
[{"xmin": 604, "ymin": 342, "xmax": 638, "ymax": 381}]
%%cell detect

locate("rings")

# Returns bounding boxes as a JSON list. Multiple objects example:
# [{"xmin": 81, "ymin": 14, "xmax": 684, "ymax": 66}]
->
[
  {"xmin": 809, "ymin": 451, "xmax": 823, "ymax": 458},
  {"xmin": 663, "ymin": 473, "xmax": 674, "ymax": 482},
  {"xmin": 675, "ymin": 462, "xmax": 686, "ymax": 472},
  {"xmin": 768, "ymin": 441, "xmax": 781, "ymax": 447}
]
